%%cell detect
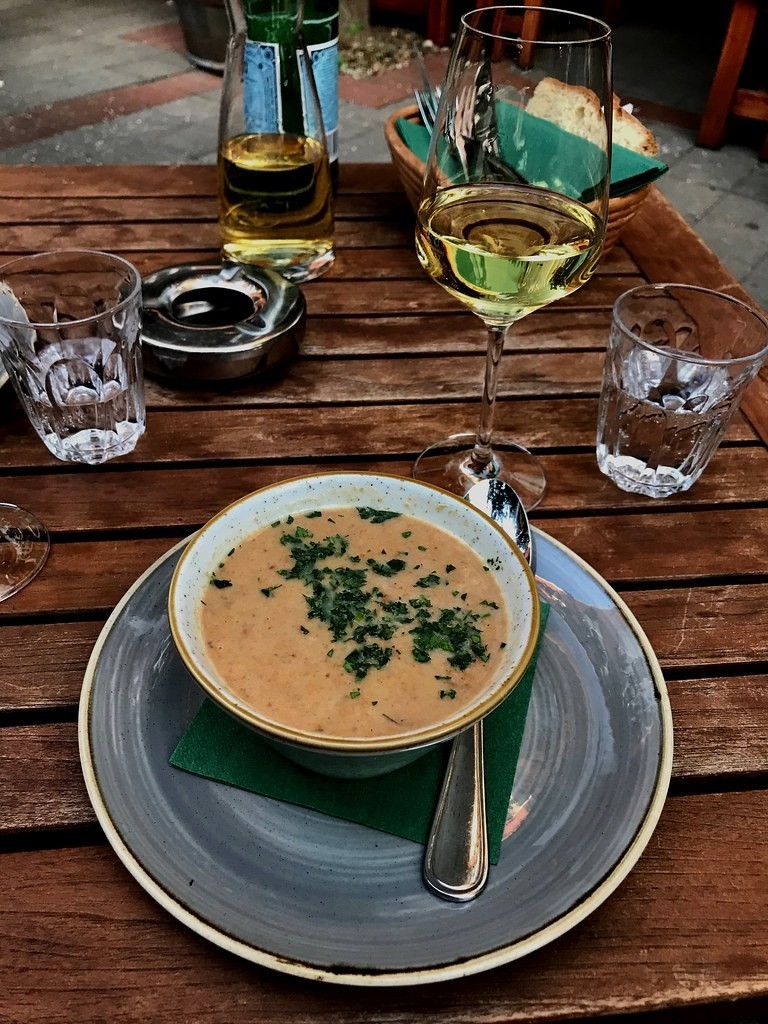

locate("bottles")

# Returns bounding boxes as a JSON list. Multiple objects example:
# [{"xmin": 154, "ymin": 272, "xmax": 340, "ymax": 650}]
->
[{"xmin": 243, "ymin": 0, "xmax": 339, "ymax": 207}]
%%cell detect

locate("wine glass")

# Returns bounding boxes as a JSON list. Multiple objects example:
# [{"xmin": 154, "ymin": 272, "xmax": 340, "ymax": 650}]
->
[
  {"xmin": 414, "ymin": 5, "xmax": 615, "ymax": 513},
  {"xmin": 0, "ymin": 500, "xmax": 49, "ymax": 605}
]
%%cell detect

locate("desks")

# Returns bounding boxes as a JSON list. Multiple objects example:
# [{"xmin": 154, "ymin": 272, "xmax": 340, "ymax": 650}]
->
[{"xmin": 0, "ymin": 165, "xmax": 768, "ymax": 1024}]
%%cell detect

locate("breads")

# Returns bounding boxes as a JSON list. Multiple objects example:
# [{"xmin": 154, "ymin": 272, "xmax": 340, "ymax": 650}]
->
[{"xmin": 529, "ymin": 76, "xmax": 661, "ymax": 159}]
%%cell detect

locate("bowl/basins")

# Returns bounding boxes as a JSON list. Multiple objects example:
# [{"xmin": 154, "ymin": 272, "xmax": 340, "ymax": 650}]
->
[
  {"xmin": 168, "ymin": 471, "xmax": 540, "ymax": 780},
  {"xmin": 0, "ymin": 280, "xmax": 36, "ymax": 395}
]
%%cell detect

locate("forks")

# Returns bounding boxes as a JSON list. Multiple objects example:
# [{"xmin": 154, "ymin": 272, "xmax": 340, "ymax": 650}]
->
[{"xmin": 414, "ymin": 84, "xmax": 532, "ymax": 185}]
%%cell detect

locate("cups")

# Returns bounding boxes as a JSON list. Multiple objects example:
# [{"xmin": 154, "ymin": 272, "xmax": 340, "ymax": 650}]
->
[
  {"xmin": 217, "ymin": 1, "xmax": 335, "ymax": 285},
  {"xmin": 596, "ymin": 282, "xmax": 768, "ymax": 499},
  {"xmin": 0, "ymin": 250, "xmax": 148, "ymax": 464}
]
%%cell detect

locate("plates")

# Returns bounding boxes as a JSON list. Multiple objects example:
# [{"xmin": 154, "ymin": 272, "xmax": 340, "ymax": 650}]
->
[{"xmin": 77, "ymin": 523, "xmax": 674, "ymax": 988}]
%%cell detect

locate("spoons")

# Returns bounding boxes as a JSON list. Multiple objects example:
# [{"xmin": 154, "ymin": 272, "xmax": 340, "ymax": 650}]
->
[{"xmin": 423, "ymin": 479, "xmax": 533, "ymax": 903}]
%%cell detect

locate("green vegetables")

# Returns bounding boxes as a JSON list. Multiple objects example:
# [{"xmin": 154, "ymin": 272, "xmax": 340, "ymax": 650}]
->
[{"xmin": 207, "ymin": 507, "xmax": 508, "ymax": 703}]
